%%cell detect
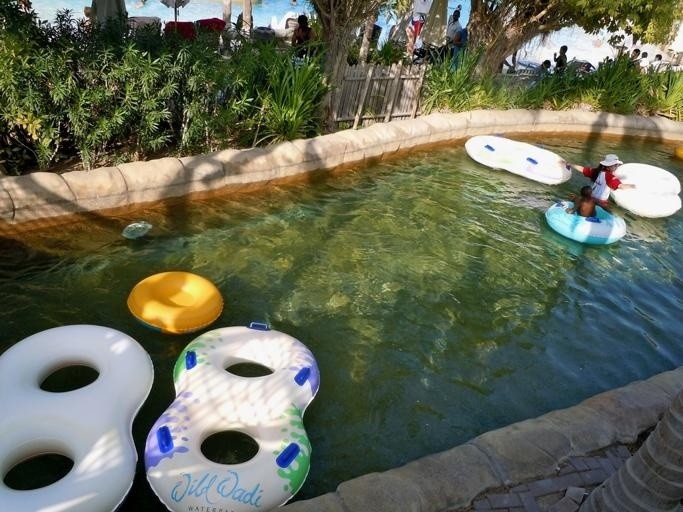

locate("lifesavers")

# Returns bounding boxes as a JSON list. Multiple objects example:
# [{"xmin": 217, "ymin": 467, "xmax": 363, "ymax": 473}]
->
[
  {"xmin": 465, "ymin": 135, "xmax": 571, "ymax": 185},
  {"xmin": 545, "ymin": 201, "xmax": 625, "ymax": 244},
  {"xmin": 127, "ymin": 271, "xmax": 224, "ymax": 334},
  {"xmin": 0, "ymin": 325, "xmax": 154, "ymax": 512},
  {"xmin": 610, "ymin": 163, "xmax": 680, "ymax": 218},
  {"xmin": 144, "ymin": 324, "xmax": 321, "ymax": 511}
]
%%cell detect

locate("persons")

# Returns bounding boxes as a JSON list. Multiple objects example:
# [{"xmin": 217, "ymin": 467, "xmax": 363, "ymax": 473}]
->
[
  {"xmin": 532, "ymin": 45, "xmax": 662, "ymax": 76},
  {"xmin": 566, "ymin": 185, "xmax": 610, "ymax": 217},
  {"xmin": 569, "ymin": 154, "xmax": 637, "ymax": 207},
  {"xmin": 292, "ymin": 5, "xmax": 467, "ymax": 69}
]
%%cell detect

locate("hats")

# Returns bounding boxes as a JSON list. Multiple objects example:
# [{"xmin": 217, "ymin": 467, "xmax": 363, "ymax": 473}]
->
[{"xmin": 599, "ymin": 154, "xmax": 622, "ymax": 166}]
